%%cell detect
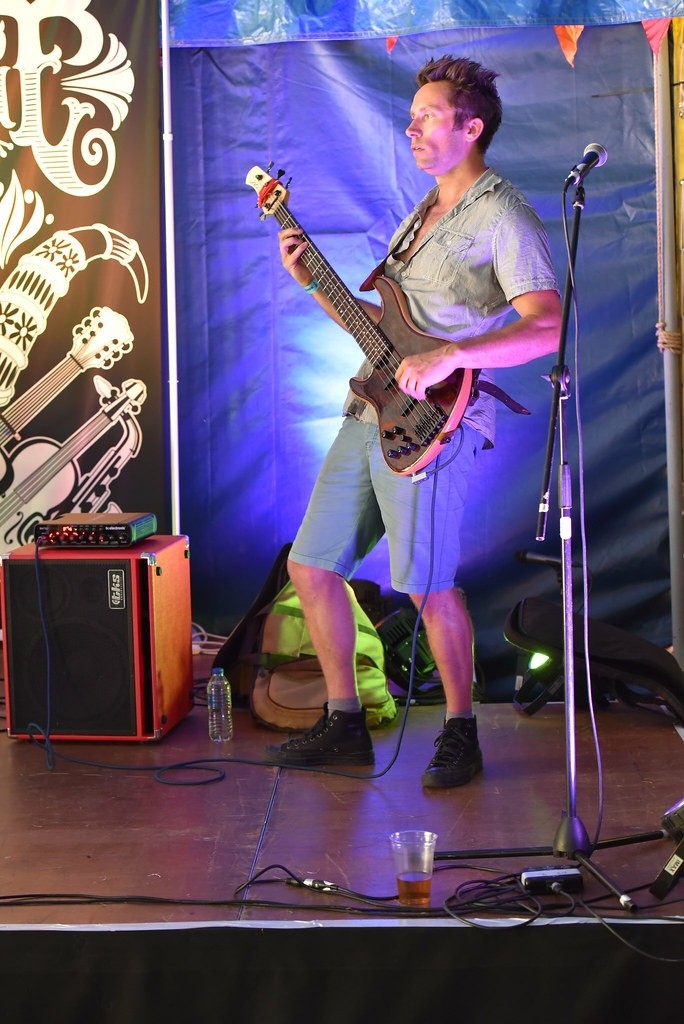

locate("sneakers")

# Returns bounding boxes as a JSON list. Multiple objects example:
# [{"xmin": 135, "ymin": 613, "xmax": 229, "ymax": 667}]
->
[
  {"xmin": 262, "ymin": 702, "xmax": 375, "ymax": 766},
  {"xmin": 422, "ymin": 714, "xmax": 483, "ymax": 788}
]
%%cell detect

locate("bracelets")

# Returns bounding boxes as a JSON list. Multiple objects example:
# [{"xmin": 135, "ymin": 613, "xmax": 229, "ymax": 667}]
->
[{"xmin": 301, "ymin": 277, "xmax": 321, "ymax": 295}]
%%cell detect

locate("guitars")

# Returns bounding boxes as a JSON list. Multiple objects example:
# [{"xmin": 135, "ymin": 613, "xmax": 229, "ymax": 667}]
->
[{"xmin": 238, "ymin": 160, "xmax": 479, "ymax": 477}]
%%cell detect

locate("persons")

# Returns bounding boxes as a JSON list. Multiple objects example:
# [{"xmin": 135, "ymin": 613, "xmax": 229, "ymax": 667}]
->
[{"xmin": 276, "ymin": 53, "xmax": 566, "ymax": 791}]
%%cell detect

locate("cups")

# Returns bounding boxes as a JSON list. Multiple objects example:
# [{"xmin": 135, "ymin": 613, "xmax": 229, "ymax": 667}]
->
[{"xmin": 389, "ymin": 830, "xmax": 439, "ymax": 905}]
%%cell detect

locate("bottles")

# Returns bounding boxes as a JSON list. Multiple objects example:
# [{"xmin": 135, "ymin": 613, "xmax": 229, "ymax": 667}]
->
[{"xmin": 206, "ymin": 667, "xmax": 233, "ymax": 742}]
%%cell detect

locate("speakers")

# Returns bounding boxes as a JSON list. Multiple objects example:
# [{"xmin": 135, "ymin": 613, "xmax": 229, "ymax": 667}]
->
[{"xmin": 0, "ymin": 534, "xmax": 192, "ymax": 740}]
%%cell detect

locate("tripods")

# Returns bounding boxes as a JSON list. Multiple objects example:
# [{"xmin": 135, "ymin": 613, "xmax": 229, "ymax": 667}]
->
[{"xmin": 432, "ymin": 182, "xmax": 675, "ymax": 912}]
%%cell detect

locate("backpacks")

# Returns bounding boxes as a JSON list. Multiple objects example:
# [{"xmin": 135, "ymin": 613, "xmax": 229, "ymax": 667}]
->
[{"xmin": 251, "ymin": 545, "xmax": 397, "ymax": 729}]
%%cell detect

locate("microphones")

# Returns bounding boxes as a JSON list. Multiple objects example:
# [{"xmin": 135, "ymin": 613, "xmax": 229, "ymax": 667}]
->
[{"xmin": 563, "ymin": 143, "xmax": 609, "ymax": 193}]
